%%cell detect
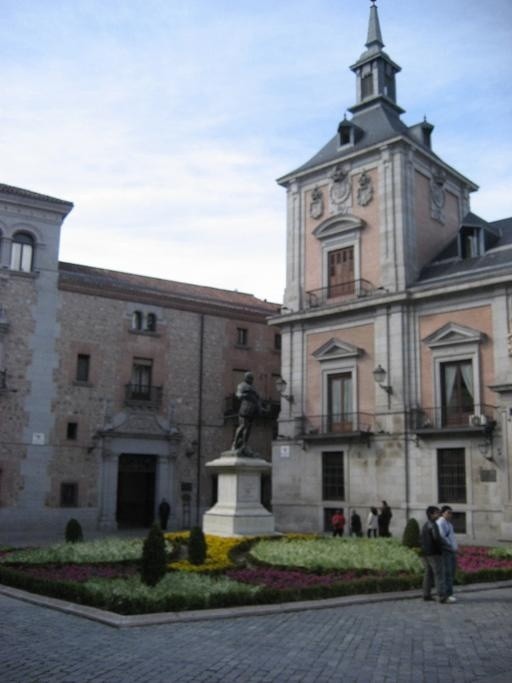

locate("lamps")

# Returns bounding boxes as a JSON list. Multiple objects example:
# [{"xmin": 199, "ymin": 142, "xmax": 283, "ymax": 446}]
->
[
  {"xmin": 373, "ymin": 364, "xmax": 392, "ymax": 394},
  {"xmin": 274, "ymin": 377, "xmax": 294, "ymax": 403}
]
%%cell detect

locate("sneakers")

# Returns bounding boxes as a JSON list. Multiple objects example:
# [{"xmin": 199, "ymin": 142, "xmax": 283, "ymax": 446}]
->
[{"xmin": 424, "ymin": 592, "xmax": 457, "ymax": 603}]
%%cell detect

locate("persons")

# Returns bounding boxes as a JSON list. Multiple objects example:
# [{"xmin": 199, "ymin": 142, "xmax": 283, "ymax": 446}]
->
[
  {"xmin": 348, "ymin": 509, "xmax": 363, "ymax": 537},
  {"xmin": 418, "ymin": 504, "xmax": 458, "ymax": 604},
  {"xmin": 377, "ymin": 499, "xmax": 392, "ymax": 537},
  {"xmin": 158, "ymin": 497, "xmax": 170, "ymax": 530},
  {"xmin": 331, "ymin": 509, "xmax": 345, "ymax": 537},
  {"xmin": 366, "ymin": 505, "xmax": 378, "ymax": 538},
  {"xmin": 228, "ymin": 370, "xmax": 269, "ymax": 452},
  {"xmin": 432, "ymin": 505, "xmax": 460, "ymax": 597}
]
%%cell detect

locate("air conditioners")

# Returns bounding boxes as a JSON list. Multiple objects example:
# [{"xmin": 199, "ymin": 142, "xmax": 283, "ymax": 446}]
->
[{"xmin": 468, "ymin": 414, "xmax": 487, "ymax": 426}]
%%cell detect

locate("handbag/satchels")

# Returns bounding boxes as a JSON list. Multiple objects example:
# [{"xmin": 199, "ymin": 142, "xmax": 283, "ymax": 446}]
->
[{"xmin": 335, "ymin": 520, "xmax": 340, "ymax": 527}]
